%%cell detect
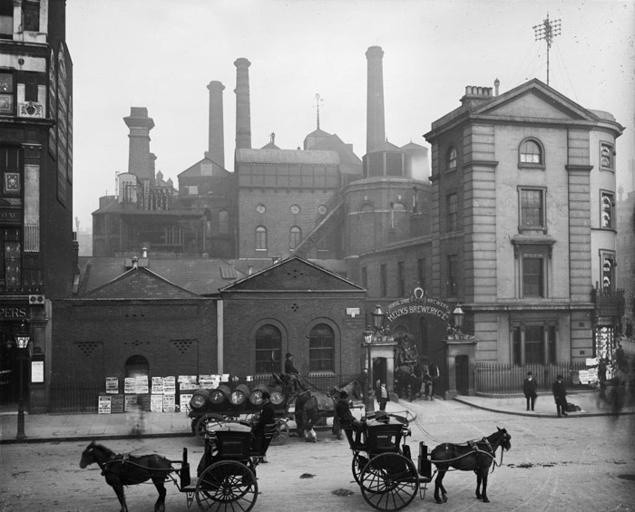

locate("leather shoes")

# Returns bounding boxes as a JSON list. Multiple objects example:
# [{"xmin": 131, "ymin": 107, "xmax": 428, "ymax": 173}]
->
[
  {"xmin": 526, "ymin": 409, "xmax": 534, "ymax": 411},
  {"xmin": 558, "ymin": 412, "xmax": 568, "ymax": 417}
]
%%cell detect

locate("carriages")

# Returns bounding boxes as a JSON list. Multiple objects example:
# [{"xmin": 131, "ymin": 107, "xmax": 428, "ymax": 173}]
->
[
  {"xmin": 351, "ymin": 411, "xmax": 510, "ymax": 511},
  {"xmin": 79, "ymin": 418, "xmax": 280, "ymax": 511},
  {"xmin": 187, "ymin": 375, "xmax": 364, "ymax": 446}
]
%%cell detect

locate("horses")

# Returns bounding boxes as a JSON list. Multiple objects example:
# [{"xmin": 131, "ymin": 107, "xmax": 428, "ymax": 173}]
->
[
  {"xmin": 431, "ymin": 426, "xmax": 511, "ymax": 505},
  {"xmin": 294, "ymin": 380, "xmax": 363, "ymax": 442},
  {"xmin": 79, "ymin": 438, "xmax": 172, "ymax": 512}
]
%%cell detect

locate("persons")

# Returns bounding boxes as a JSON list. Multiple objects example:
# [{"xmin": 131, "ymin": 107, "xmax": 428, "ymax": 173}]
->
[
  {"xmin": 255, "ymin": 391, "xmax": 277, "ymax": 453},
  {"xmin": 284, "ymin": 350, "xmax": 302, "ymax": 392},
  {"xmin": 295, "ymin": 392, "xmax": 316, "ymax": 437},
  {"xmin": 552, "ymin": 373, "xmax": 569, "ymax": 417},
  {"xmin": 377, "ymin": 382, "xmax": 390, "ymax": 412},
  {"xmin": 401, "ymin": 334, "xmax": 413, "ymax": 358},
  {"xmin": 251, "ymin": 411, "xmax": 269, "ymax": 466},
  {"xmin": 374, "ymin": 375, "xmax": 383, "ymax": 402},
  {"xmin": 299, "ymin": 389, "xmax": 321, "ymax": 441},
  {"xmin": 333, "ymin": 390, "xmax": 366, "ymax": 450},
  {"xmin": 523, "ymin": 370, "xmax": 538, "ymax": 411},
  {"xmin": 394, "ymin": 357, "xmax": 442, "ymax": 403},
  {"xmin": 360, "ymin": 383, "xmax": 375, "ymax": 412},
  {"xmin": 356, "ymin": 367, "xmax": 370, "ymax": 398},
  {"xmin": 616, "ymin": 344, "xmax": 626, "ymax": 369}
]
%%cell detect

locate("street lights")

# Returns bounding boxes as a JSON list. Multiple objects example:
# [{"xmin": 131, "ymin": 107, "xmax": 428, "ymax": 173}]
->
[
  {"xmin": 364, "ymin": 332, "xmax": 375, "ymax": 410},
  {"xmin": 16, "ymin": 334, "xmax": 29, "ymax": 440}
]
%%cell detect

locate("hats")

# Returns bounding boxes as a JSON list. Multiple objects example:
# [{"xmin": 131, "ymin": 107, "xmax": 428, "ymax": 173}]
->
[
  {"xmin": 340, "ymin": 391, "xmax": 348, "ymax": 398},
  {"xmin": 527, "ymin": 371, "xmax": 533, "ymax": 376},
  {"xmin": 556, "ymin": 375, "xmax": 563, "ymax": 379},
  {"xmin": 285, "ymin": 353, "xmax": 293, "ymax": 358}
]
